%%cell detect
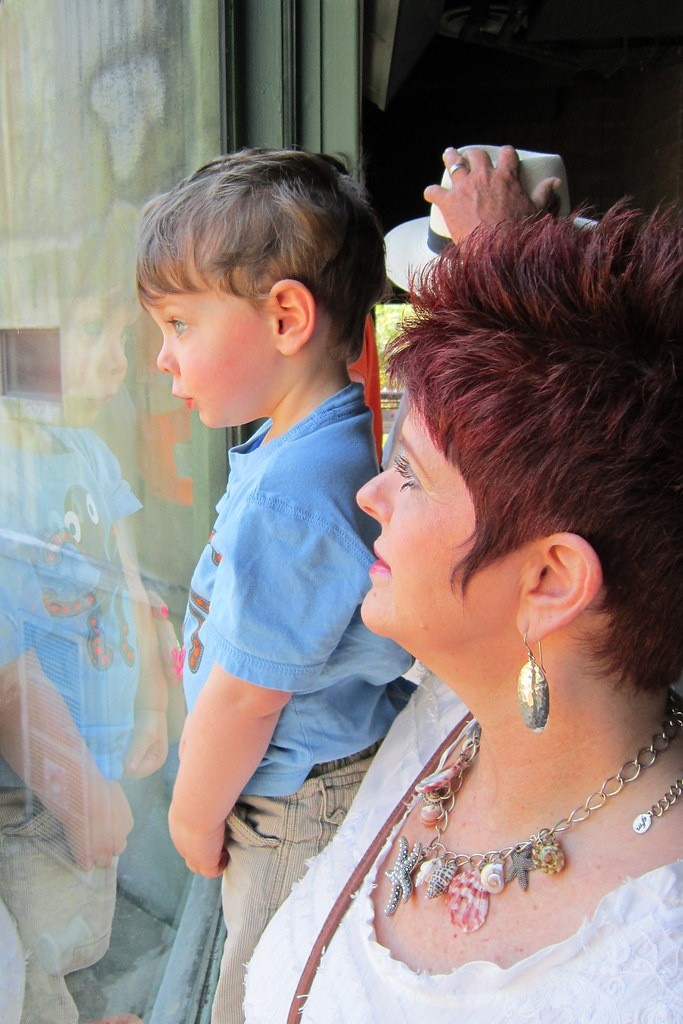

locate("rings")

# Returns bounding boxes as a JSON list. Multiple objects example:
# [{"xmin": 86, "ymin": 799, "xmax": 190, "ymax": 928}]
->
[{"xmin": 449, "ymin": 163, "xmax": 470, "ymax": 177}]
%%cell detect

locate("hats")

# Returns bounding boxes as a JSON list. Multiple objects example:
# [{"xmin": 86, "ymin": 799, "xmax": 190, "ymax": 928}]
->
[{"xmin": 384, "ymin": 146, "xmax": 601, "ymax": 299}]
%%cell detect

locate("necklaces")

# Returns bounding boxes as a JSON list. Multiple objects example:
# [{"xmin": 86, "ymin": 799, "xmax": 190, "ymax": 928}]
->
[{"xmin": 383, "ymin": 682, "xmax": 683, "ymax": 934}]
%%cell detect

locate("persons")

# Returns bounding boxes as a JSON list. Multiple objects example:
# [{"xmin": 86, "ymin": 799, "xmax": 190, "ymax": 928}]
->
[
  {"xmin": 135, "ymin": 146, "xmax": 417, "ymax": 1024},
  {"xmin": 0, "ymin": 152, "xmax": 170, "ymax": 1024},
  {"xmin": 424, "ymin": 143, "xmax": 561, "ymax": 266},
  {"xmin": 239, "ymin": 200, "xmax": 683, "ymax": 1024}
]
